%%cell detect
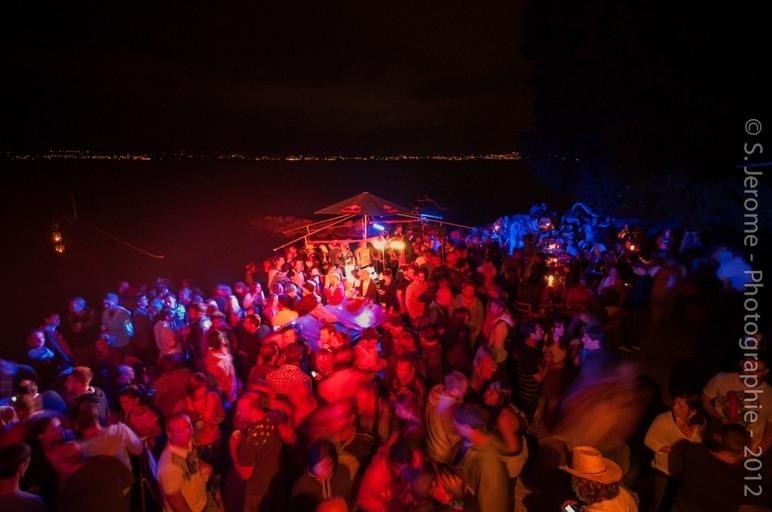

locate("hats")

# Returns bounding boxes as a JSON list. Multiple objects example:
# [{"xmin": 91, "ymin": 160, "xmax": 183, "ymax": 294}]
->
[{"xmin": 559, "ymin": 445, "xmax": 623, "ymax": 484}]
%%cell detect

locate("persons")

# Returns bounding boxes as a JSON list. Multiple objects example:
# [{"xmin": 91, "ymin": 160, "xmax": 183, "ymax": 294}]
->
[{"xmin": 0, "ymin": 199, "xmax": 770, "ymax": 510}]
